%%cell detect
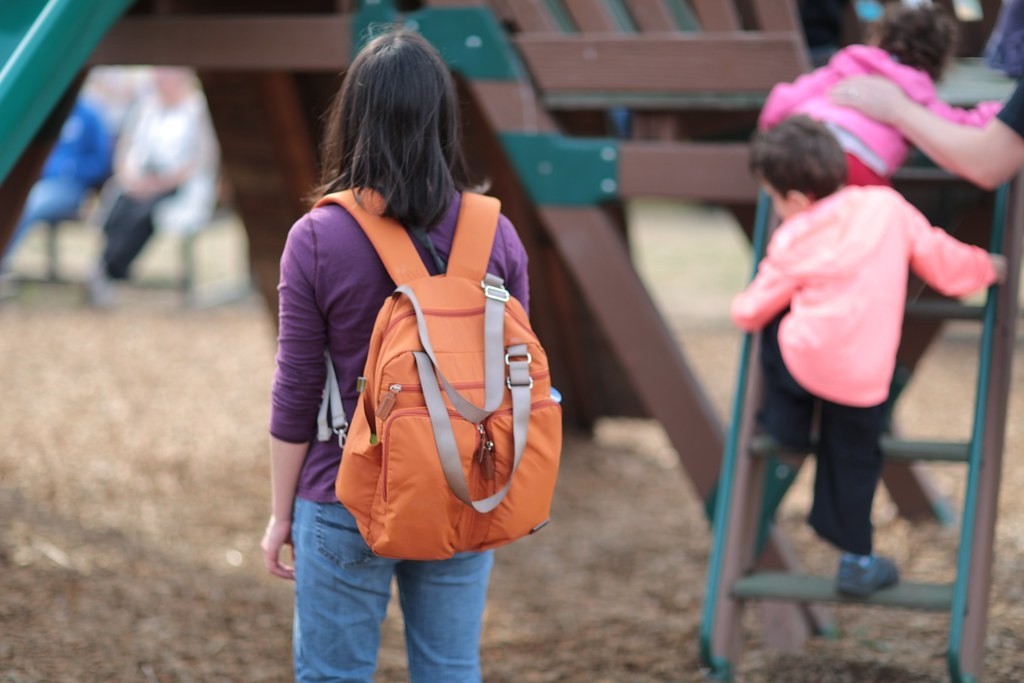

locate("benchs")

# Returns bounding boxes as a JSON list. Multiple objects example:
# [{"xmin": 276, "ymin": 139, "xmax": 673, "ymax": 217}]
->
[{"xmin": 0, "ymin": 180, "xmax": 256, "ymax": 306}]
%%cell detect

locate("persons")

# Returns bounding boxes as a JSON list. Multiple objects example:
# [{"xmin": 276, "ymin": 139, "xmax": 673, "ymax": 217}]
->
[
  {"xmin": 757, "ymin": 0, "xmax": 1004, "ymax": 188},
  {"xmin": 262, "ymin": 31, "xmax": 529, "ymax": 683},
  {"xmin": 15, "ymin": 67, "xmax": 218, "ymax": 303},
  {"xmin": 731, "ymin": 114, "xmax": 1009, "ymax": 599},
  {"xmin": 828, "ymin": 74, "xmax": 1024, "ymax": 189}
]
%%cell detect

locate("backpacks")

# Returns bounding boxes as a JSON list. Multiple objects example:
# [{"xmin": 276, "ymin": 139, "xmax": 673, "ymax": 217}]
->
[{"xmin": 308, "ymin": 186, "xmax": 564, "ymax": 562}]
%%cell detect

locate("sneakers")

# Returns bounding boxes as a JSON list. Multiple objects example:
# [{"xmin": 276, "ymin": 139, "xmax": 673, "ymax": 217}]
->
[{"xmin": 833, "ymin": 552, "xmax": 900, "ymax": 597}]
